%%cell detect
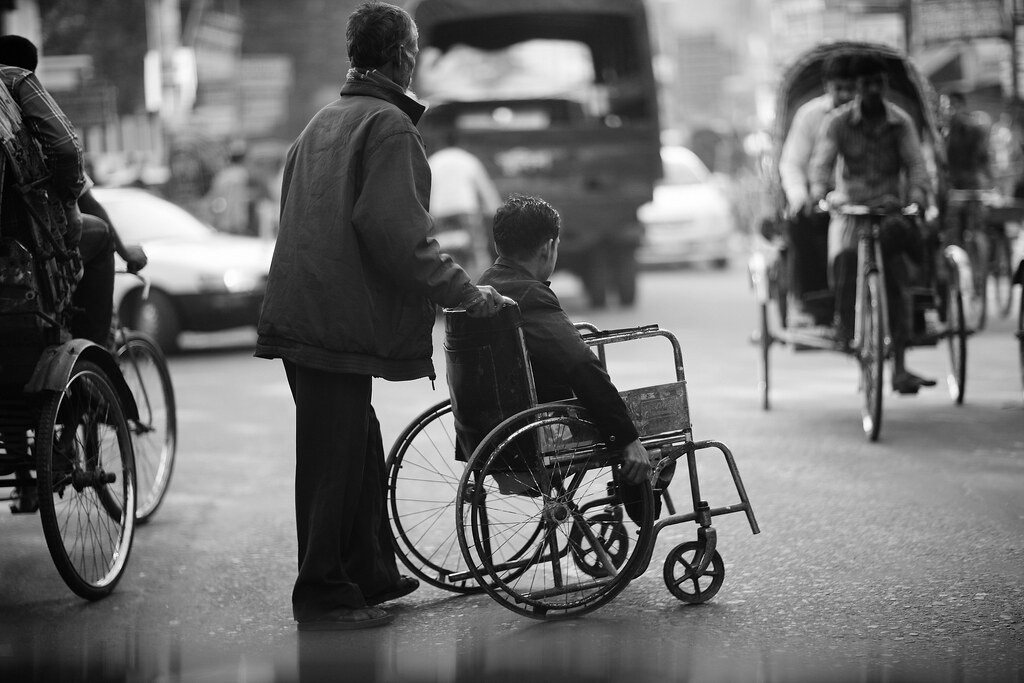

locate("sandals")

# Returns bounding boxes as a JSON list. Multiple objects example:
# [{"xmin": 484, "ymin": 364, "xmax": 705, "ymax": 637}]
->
[
  {"xmin": 366, "ymin": 574, "xmax": 419, "ymax": 605},
  {"xmin": 299, "ymin": 606, "xmax": 395, "ymax": 630}
]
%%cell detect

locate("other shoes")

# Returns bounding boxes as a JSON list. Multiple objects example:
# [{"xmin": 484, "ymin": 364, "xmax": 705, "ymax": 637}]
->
[{"xmin": 895, "ymin": 376, "xmax": 938, "ymax": 393}]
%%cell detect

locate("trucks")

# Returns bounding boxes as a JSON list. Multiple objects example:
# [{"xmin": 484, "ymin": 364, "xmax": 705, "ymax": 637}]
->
[{"xmin": 406, "ymin": 1, "xmax": 664, "ymax": 307}]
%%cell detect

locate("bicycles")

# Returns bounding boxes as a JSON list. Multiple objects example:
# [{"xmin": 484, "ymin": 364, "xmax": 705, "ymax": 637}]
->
[{"xmin": 944, "ymin": 194, "xmax": 1023, "ymax": 332}]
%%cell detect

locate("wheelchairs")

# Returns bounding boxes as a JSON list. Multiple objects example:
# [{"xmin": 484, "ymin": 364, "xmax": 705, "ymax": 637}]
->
[{"xmin": 385, "ymin": 295, "xmax": 760, "ymax": 620}]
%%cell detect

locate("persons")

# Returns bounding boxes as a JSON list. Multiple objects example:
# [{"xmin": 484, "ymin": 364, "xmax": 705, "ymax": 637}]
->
[
  {"xmin": 0, "ymin": 32, "xmax": 148, "ymax": 343},
  {"xmin": 252, "ymin": 0, "xmax": 507, "ymax": 632},
  {"xmin": 779, "ymin": 49, "xmax": 937, "ymax": 395},
  {"xmin": 448, "ymin": 194, "xmax": 677, "ymax": 531},
  {"xmin": 203, "ymin": 139, "xmax": 278, "ymax": 236},
  {"xmin": 933, "ymin": 89, "xmax": 995, "ymax": 249},
  {"xmin": 420, "ymin": 130, "xmax": 509, "ymax": 236}
]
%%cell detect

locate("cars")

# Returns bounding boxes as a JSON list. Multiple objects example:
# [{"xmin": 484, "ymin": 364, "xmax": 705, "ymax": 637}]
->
[
  {"xmin": 636, "ymin": 146, "xmax": 736, "ymax": 271},
  {"xmin": 91, "ymin": 187, "xmax": 270, "ymax": 353}
]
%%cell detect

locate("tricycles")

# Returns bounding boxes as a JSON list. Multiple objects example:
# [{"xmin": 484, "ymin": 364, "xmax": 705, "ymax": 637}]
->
[
  {"xmin": 752, "ymin": 40, "xmax": 970, "ymax": 442},
  {"xmin": 0, "ymin": 84, "xmax": 177, "ymax": 600}
]
